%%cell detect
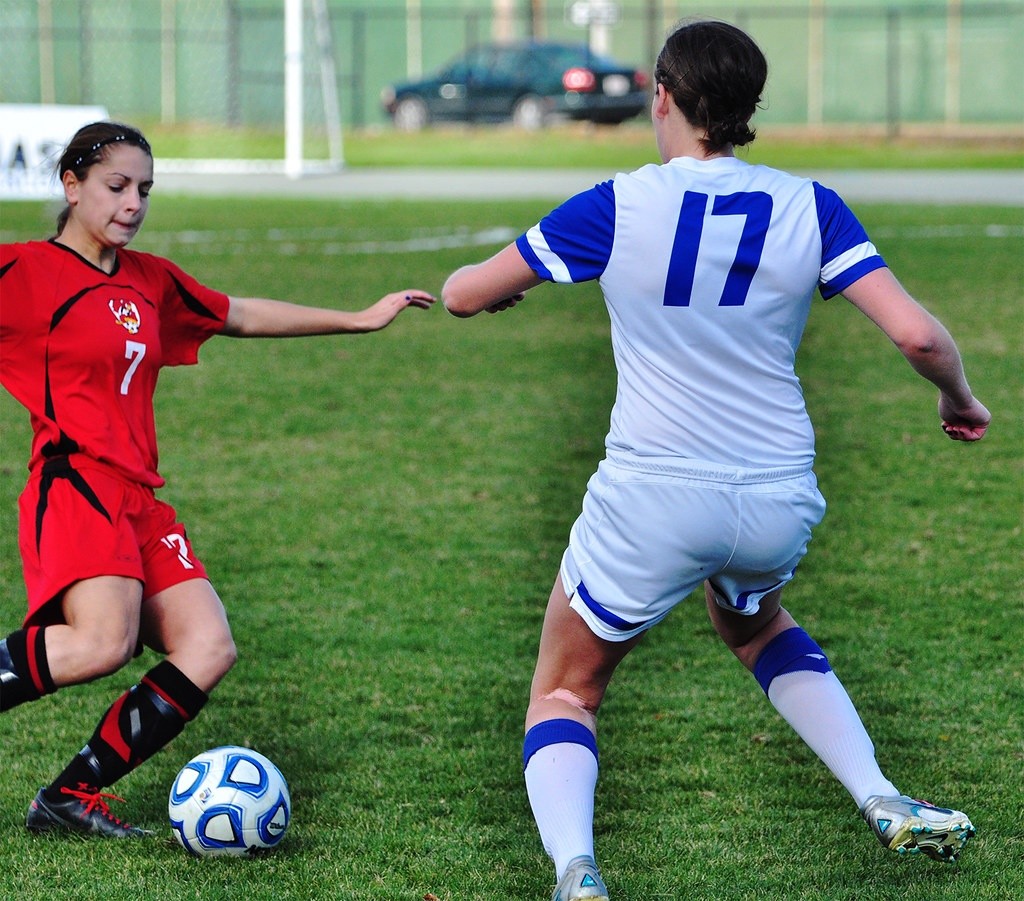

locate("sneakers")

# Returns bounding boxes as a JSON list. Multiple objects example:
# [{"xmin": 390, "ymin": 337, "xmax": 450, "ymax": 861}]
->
[
  {"xmin": 26, "ymin": 787, "xmax": 151, "ymax": 840},
  {"xmin": 858, "ymin": 794, "xmax": 975, "ymax": 863},
  {"xmin": 551, "ymin": 855, "xmax": 610, "ymax": 901}
]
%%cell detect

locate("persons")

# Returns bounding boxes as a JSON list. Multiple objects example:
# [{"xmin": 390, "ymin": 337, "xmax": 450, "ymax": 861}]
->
[
  {"xmin": 435, "ymin": 21, "xmax": 992, "ymax": 901},
  {"xmin": 0, "ymin": 123, "xmax": 437, "ymax": 843}
]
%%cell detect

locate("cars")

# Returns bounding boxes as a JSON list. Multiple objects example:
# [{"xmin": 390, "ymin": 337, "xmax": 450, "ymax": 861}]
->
[{"xmin": 381, "ymin": 41, "xmax": 650, "ymax": 131}]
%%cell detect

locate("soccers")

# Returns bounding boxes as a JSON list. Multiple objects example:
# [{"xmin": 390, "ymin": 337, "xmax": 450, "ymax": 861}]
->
[{"xmin": 168, "ymin": 744, "xmax": 291, "ymax": 862}]
